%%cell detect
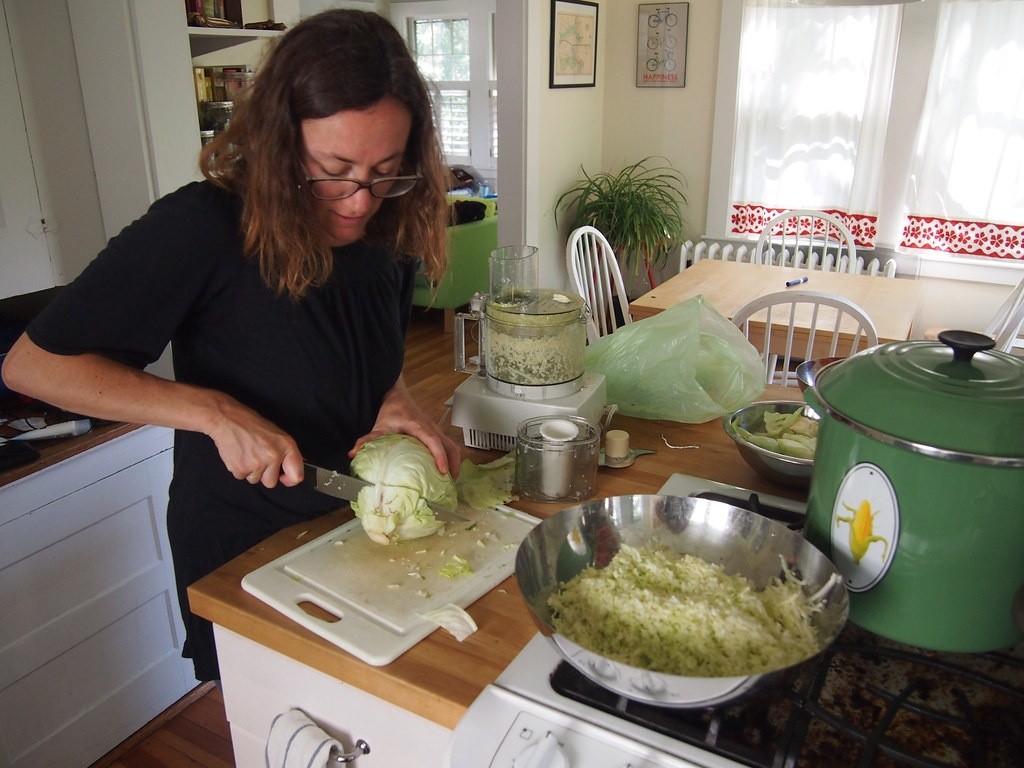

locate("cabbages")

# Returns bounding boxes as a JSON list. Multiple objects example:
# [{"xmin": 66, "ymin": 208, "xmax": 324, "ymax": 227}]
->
[
  {"xmin": 732, "ymin": 407, "xmax": 819, "ymax": 461},
  {"xmin": 347, "ymin": 433, "xmax": 519, "ymax": 546}
]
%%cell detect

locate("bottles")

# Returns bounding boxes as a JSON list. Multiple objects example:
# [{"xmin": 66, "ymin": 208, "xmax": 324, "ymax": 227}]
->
[{"xmin": 199, "ymin": 72, "xmax": 255, "ymax": 172}]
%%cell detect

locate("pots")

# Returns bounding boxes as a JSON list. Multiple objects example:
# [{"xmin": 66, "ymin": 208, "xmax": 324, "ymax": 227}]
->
[
  {"xmin": 797, "ymin": 328, "xmax": 1024, "ymax": 655},
  {"xmin": 514, "ymin": 491, "xmax": 850, "ymax": 708}
]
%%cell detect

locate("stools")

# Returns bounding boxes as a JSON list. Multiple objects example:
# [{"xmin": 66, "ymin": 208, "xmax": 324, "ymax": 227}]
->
[{"xmin": 589, "ymin": 241, "xmax": 668, "ymax": 331}]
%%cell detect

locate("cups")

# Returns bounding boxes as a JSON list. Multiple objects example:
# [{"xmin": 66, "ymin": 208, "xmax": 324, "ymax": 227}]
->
[{"xmin": 513, "ymin": 414, "xmax": 601, "ymax": 505}]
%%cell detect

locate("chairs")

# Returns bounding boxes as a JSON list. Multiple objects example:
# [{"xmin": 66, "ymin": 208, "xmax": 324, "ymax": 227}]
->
[
  {"xmin": 755, "ymin": 210, "xmax": 855, "ymax": 275},
  {"xmin": 566, "ymin": 226, "xmax": 632, "ymax": 344},
  {"xmin": 983, "ymin": 276, "xmax": 1024, "ymax": 353},
  {"xmin": 410, "ymin": 194, "xmax": 499, "ymax": 333},
  {"xmin": 731, "ymin": 289, "xmax": 878, "ymax": 392}
]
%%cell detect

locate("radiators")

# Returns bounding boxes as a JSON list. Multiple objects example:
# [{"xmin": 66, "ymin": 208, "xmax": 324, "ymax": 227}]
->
[{"xmin": 680, "ymin": 239, "xmax": 897, "ymax": 279}]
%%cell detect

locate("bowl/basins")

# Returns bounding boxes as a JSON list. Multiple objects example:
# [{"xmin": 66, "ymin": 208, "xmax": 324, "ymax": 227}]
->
[{"xmin": 725, "ymin": 399, "xmax": 826, "ymax": 488}]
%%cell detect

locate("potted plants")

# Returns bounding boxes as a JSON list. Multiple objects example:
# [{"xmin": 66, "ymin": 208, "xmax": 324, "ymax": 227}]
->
[{"xmin": 553, "ymin": 154, "xmax": 688, "ymax": 275}]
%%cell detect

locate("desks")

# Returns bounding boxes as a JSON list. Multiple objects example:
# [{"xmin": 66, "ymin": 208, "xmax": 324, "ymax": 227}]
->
[
  {"xmin": 628, "ymin": 258, "xmax": 924, "ymax": 364},
  {"xmin": 185, "ymin": 378, "xmax": 1024, "ymax": 768}
]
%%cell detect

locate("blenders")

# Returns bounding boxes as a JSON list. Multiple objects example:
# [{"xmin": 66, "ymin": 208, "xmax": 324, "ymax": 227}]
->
[{"xmin": 451, "ymin": 244, "xmax": 607, "ymax": 455}]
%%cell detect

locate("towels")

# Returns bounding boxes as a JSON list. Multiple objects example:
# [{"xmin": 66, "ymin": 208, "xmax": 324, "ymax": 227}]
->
[{"xmin": 266, "ymin": 709, "xmax": 346, "ymax": 768}]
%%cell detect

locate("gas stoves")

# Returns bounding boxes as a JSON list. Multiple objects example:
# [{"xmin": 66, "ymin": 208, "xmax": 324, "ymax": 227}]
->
[{"xmin": 438, "ymin": 474, "xmax": 1023, "ymax": 768}]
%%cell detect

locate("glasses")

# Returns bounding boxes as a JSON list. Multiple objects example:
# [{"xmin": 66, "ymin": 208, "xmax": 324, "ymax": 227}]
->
[{"xmin": 291, "ymin": 154, "xmax": 423, "ymax": 200}]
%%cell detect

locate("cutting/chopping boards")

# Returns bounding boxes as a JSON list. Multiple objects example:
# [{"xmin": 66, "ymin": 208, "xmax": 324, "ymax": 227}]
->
[{"xmin": 241, "ymin": 499, "xmax": 545, "ymax": 667}]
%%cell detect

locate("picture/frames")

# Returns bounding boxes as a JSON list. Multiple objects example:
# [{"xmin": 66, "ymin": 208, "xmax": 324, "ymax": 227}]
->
[
  {"xmin": 549, "ymin": 0, "xmax": 598, "ymax": 89},
  {"xmin": 636, "ymin": 2, "xmax": 689, "ymax": 88}
]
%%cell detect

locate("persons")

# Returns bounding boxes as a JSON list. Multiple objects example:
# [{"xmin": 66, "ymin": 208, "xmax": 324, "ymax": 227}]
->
[{"xmin": 0, "ymin": 9, "xmax": 462, "ymax": 690}]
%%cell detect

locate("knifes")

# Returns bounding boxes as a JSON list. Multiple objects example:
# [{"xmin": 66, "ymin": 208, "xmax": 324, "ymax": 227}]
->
[{"xmin": 298, "ymin": 457, "xmax": 470, "ymax": 524}]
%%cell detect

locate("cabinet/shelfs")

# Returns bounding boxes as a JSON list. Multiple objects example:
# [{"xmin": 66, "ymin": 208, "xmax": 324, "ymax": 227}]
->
[{"xmin": 0, "ymin": 423, "xmax": 216, "ymax": 768}]
